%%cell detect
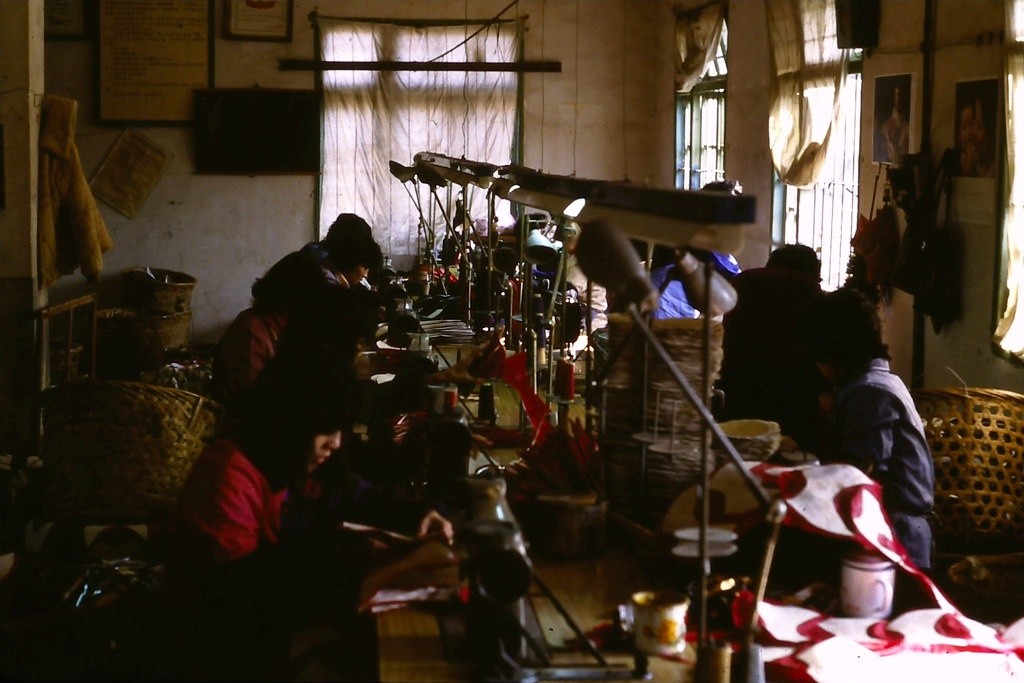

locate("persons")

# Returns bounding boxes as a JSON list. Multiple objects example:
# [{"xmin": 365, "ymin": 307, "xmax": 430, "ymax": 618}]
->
[
  {"xmin": 958, "ymin": 79, "xmax": 995, "ymax": 177},
  {"xmin": 649, "ymin": 181, "xmax": 935, "ymax": 575},
  {"xmin": 168, "ymin": 212, "xmax": 491, "ymax": 631},
  {"xmin": 874, "ymin": 84, "xmax": 909, "ymax": 163}
]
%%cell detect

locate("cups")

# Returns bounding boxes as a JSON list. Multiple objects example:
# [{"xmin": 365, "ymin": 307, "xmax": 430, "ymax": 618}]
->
[
  {"xmin": 629, "ymin": 588, "xmax": 692, "ymax": 657},
  {"xmin": 836, "ymin": 552, "xmax": 898, "ymax": 621}
]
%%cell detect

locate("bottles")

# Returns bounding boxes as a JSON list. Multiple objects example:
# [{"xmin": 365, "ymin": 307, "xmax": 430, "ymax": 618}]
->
[
  {"xmin": 440, "ymin": 389, "xmax": 463, "ymax": 421},
  {"xmin": 676, "ymin": 249, "xmax": 739, "ymax": 314},
  {"xmin": 477, "ymin": 382, "xmax": 497, "ymax": 424}
]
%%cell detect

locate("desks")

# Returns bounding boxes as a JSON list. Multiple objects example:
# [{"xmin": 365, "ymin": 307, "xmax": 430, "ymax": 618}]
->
[{"xmin": 376, "ymin": 346, "xmax": 696, "ymax": 683}]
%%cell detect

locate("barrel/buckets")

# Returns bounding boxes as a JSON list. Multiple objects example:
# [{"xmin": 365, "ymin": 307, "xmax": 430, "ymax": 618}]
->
[
  {"xmin": 92, "ymin": 307, "xmax": 194, "ymax": 377},
  {"xmin": 133, "ymin": 268, "xmax": 196, "ymax": 314}
]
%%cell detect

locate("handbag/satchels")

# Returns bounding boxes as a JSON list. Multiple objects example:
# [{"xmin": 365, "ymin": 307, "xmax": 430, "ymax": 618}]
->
[{"xmin": 849, "ymin": 146, "xmax": 967, "ymax": 328}]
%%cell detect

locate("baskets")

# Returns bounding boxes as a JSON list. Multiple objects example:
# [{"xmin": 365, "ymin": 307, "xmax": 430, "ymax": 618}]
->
[
  {"xmin": 39, "ymin": 376, "xmax": 228, "ymax": 517},
  {"xmin": 911, "ymin": 362, "xmax": 1024, "ymax": 545},
  {"xmin": 94, "ymin": 264, "xmax": 199, "ymax": 375}
]
